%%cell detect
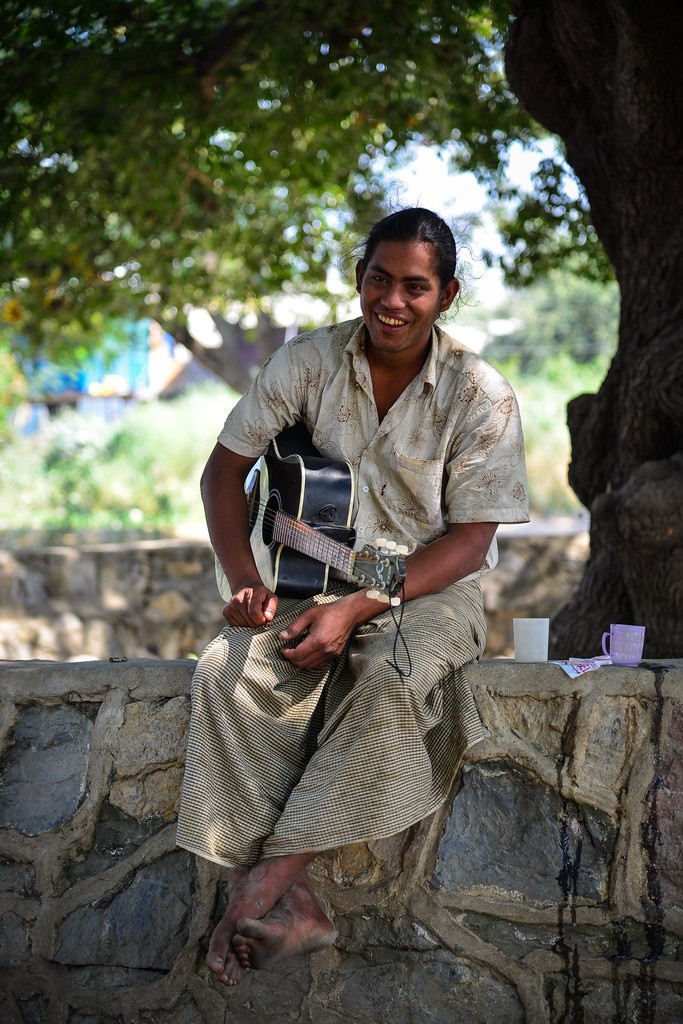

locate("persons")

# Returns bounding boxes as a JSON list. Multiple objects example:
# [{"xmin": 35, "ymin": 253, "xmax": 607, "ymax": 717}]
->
[{"xmin": 176, "ymin": 207, "xmax": 530, "ymax": 987}]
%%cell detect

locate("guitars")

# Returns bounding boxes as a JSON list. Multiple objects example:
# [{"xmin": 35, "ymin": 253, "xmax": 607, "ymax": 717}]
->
[{"xmin": 214, "ymin": 422, "xmax": 410, "ymax": 606}]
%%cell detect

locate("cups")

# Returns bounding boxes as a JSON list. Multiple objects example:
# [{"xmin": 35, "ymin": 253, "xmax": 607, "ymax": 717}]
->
[
  {"xmin": 602, "ymin": 625, "xmax": 646, "ymax": 668},
  {"xmin": 512, "ymin": 618, "xmax": 549, "ymax": 664}
]
